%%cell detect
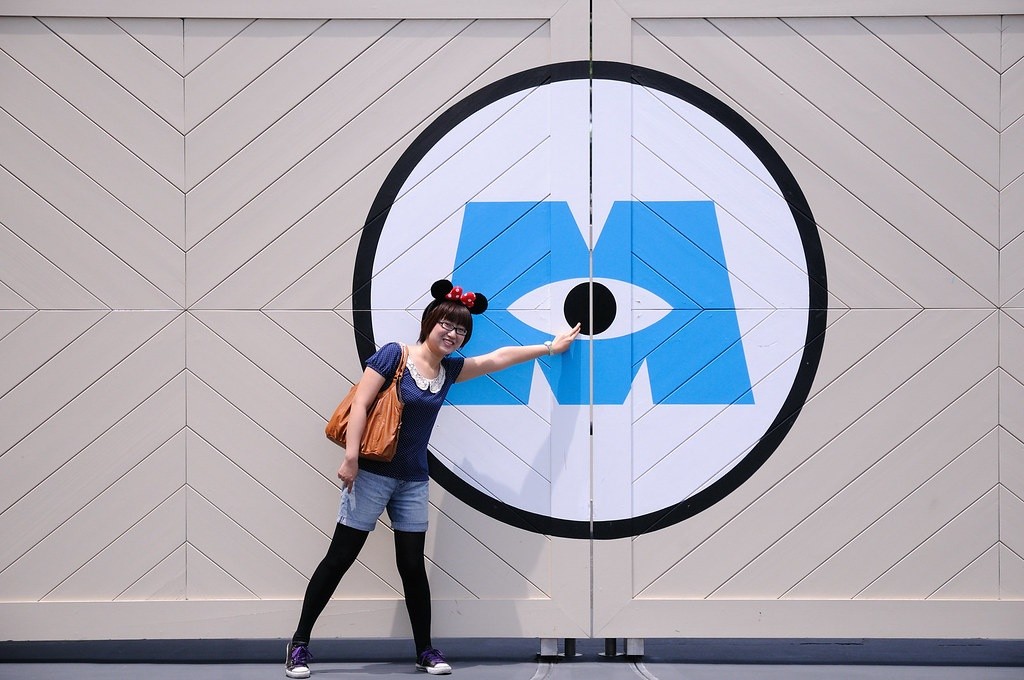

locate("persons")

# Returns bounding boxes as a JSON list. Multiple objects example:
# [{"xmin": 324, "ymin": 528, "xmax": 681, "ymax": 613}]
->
[{"xmin": 285, "ymin": 280, "xmax": 581, "ymax": 678}]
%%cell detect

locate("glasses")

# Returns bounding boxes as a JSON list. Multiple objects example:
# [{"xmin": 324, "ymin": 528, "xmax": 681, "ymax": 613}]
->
[{"xmin": 437, "ymin": 321, "xmax": 467, "ymax": 336}]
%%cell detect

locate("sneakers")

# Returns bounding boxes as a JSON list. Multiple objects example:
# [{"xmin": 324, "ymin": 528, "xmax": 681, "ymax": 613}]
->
[
  {"xmin": 286, "ymin": 640, "xmax": 314, "ymax": 677},
  {"xmin": 415, "ymin": 648, "xmax": 452, "ymax": 674}
]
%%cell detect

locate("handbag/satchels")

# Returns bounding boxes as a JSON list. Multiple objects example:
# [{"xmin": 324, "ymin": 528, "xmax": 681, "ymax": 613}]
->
[{"xmin": 325, "ymin": 342, "xmax": 407, "ymax": 461}]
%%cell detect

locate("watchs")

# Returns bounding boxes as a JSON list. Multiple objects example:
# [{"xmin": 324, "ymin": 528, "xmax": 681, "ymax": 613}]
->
[{"xmin": 545, "ymin": 340, "xmax": 554, "ymax": 356}]
